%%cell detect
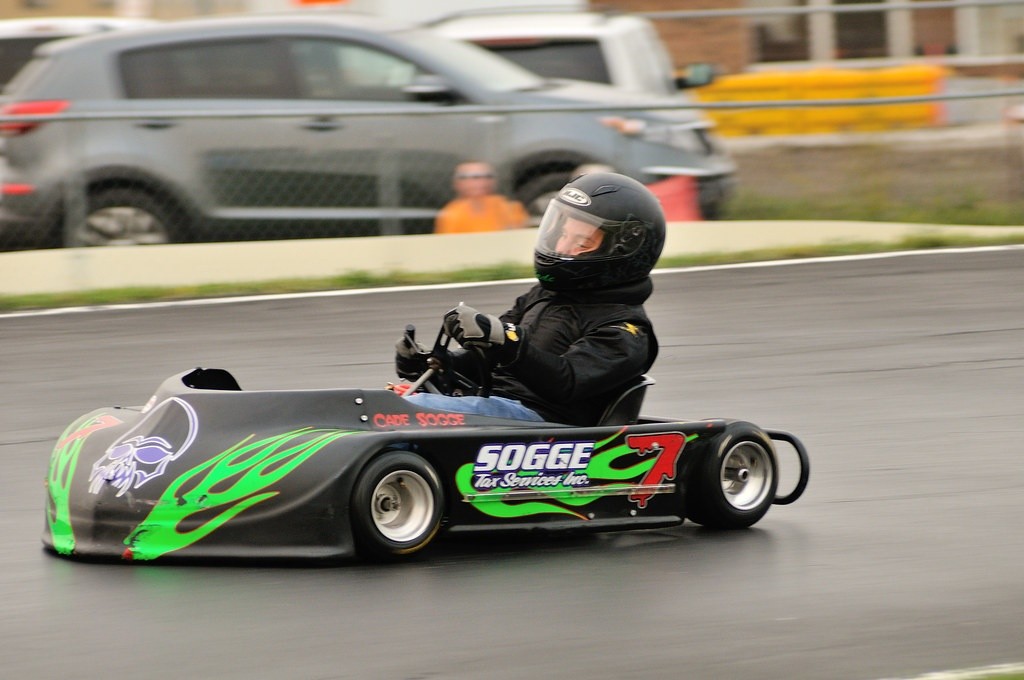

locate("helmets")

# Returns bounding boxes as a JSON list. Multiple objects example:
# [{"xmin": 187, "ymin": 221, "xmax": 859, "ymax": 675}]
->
[{"xmin": 533, "ymin": 171, "xmax": 666, "ymax": 293}]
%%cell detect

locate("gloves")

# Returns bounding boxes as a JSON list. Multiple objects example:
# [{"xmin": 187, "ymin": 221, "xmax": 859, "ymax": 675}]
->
[
  {"xmin": 395, "ymin": 339, "xmax": 425, "ymax": 382},
  {"xmin": 442, "ymin": 305, "xmax": 529, "ymax": 368}
]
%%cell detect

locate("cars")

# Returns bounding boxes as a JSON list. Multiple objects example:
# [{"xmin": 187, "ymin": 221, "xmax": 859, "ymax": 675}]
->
[
  {"xmin": 0, "ymin": 15, "xmax": 741, "ymax": 254},
  {"xmin": 432, "ymin": 15, "xmax": 680, "ymax": 101}
]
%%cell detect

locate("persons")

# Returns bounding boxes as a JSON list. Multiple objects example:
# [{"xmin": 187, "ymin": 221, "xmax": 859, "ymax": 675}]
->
[
  {"xmin": 394, "ymin": 170, "xmax": 666, "ymax": 423},
  {"xmin": 433, "ymin": 160, "xmax": 527, "ymax": 232}
]
